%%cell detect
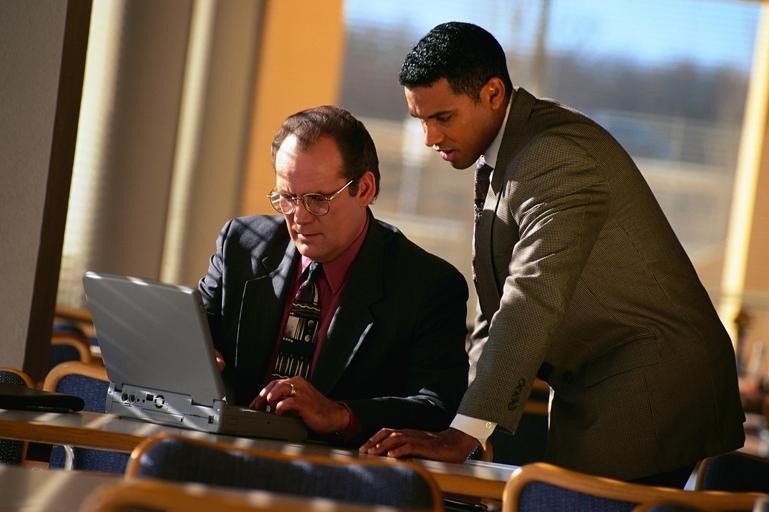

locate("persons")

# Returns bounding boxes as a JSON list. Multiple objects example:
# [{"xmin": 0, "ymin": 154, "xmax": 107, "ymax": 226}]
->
[
  {"xmin": 356, "ymin": 20, "xmax": 747, "ymax": 509},
  {"xmin": 195, "ymin": 105, "xmax": 470, "ymax": 439}
]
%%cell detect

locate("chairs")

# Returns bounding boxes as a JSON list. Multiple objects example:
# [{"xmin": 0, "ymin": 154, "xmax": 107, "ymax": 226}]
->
[{"xmin": 2, "ymin": 303, "xmax": 769, "ymax": 510}]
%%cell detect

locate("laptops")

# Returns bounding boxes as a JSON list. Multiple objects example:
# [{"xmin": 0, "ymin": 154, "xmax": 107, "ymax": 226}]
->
[{"xmin": 82, "ymin": 270, "xmax": 334, "ymax": 445}]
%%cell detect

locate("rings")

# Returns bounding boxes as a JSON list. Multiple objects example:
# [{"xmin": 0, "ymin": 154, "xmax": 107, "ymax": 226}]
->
[{"xmin": 290, "ymin": 382, "xmax": 297, "ymax": 398}]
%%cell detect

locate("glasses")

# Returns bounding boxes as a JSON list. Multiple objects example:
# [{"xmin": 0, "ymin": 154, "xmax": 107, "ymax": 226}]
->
[{"xmin": 266, "ymin": 180, "xmax": 353, "ymax": 216}]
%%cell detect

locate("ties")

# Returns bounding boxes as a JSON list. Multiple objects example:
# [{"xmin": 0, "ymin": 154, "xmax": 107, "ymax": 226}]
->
[
  {"xmin": 474, "ymin": 162, "xmax": 493, "ymax": 224},
  {"xmin": 265, "ymin": 261, "xmax": 324, "ymax": 419}
]
